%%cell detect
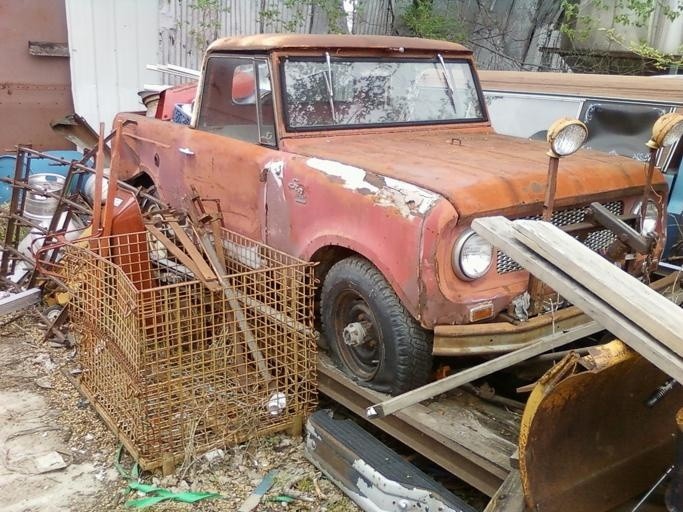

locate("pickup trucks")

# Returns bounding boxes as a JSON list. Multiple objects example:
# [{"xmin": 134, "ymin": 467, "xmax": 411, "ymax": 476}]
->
[{"xmin": 110, "ymin": 32, "xmax": 667, "ymax": 398}]
